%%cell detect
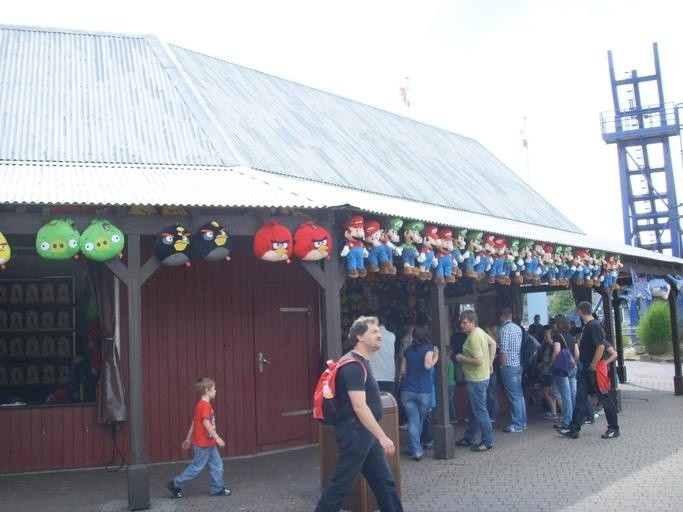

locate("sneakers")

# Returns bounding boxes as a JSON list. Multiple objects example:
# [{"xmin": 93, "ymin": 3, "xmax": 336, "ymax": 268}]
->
[
  {"xmin": 210, "ymin": 488, "xmax": 232, "ymax": 496},
  {"xmin": 399, "ymin": 399, "xmax": 620, "ymax": 461},
  {"xmin": 167, "ymin": 481, "xmax": 184, "ymax": 498}
]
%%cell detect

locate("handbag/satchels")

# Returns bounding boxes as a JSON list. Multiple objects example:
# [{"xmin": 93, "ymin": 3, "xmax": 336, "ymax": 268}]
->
[
  {"xmin": 521, "ymin": 333, "xmax": 544, "ymax": 365},
  {"xmin": 567, "ymin": 354, "xmax": 575, "ymax": 369}
]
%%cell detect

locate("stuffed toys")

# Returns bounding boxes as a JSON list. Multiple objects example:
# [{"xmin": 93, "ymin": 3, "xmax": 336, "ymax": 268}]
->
[
  {"xmin": 0, "ymin": 228, "xmax": 12, "ymax": 269},
  {"xmin": 339, "ymin": 216, "xmax": 683, "ymax": 310},
  {"xmin": 338, "ymin": 215, "xmax": 683, "ymax": 311},
  {"xmin": 36, "ymin": 217, "xmax": 81, "ymax": 260},
  {"xmin": 154, "ymin": 223, "xmax": 195, "ymax": 268},
  {"xmin": 292, "ymin": 220, "xmax": 334, "ymax": 262},
  {"xmin": 253, "ymin": 218, "xmax": 295, "ymax": 265},
  {"xmin": 80, "ymin": 217, "xmax": 125, "ymax": 263},
  {"xmin": 196, "ymin": 220, "xmax": 234, "ymax": 262}
]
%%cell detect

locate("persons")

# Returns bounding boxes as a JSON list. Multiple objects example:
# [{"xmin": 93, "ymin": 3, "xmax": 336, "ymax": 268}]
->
[
  {"xmin": 365, "ymin": 299, "xmax": 623, "ymax": 461},
  {"xmin": 165, "ymin": 375, "xmax": 232, "ymax": 500},
  {"xmin": 315, "ymin": 316, "xmax": 404, "ymax": 512}
]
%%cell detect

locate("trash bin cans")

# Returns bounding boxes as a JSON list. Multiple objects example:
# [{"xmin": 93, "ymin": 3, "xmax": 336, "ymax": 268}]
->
[{"xmin": 319, "ymin": 391, "xmax": 401, "ymax": 512}]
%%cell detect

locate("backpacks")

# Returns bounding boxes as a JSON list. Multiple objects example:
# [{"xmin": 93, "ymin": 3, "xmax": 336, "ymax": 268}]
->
[{"xmin": 313, "ymin": 356, "xmax": 368, "ymax": 425}]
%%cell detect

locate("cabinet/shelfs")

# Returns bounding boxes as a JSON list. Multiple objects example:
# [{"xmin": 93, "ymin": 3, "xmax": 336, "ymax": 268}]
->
[{"xmin": 0, "ymin": 275, "xmax": 76, "ymax": 387}]
